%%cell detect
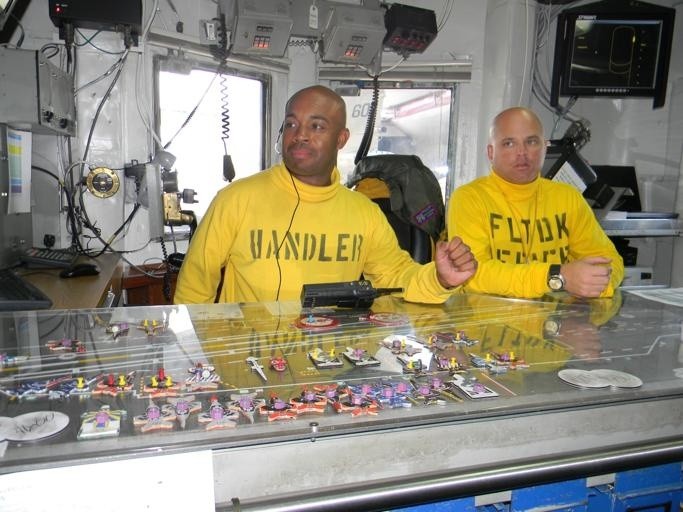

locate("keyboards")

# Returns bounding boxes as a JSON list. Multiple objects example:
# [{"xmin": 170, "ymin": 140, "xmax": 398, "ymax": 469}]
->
[{"xmin": 0, "ymin": 268, "xmax": 54, "ymax": 310}]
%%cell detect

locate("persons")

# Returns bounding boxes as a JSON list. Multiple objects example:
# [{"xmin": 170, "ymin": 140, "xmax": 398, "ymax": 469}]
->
[
  {"xmin": 174, "ymin": 87, "xmax": 478, "ymax": 303},
  {"xmin": 447, "ymin": 106, "xmax": 624, "ymax": 298}
]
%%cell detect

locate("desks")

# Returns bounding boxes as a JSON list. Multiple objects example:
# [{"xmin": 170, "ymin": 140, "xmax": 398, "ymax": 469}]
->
[{"xmin": 8, "ymin": 246, "xmax": 127, "ymax": 307}]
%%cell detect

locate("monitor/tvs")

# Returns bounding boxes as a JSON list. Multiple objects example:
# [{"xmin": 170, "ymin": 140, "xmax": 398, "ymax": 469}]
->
[{"xmin": 563, "ymin": 12, "xmax": 664, "ymax": 98}]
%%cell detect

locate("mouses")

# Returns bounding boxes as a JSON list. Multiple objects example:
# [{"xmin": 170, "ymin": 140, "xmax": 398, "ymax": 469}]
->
[{"xmin": 61, "ymin": 262, "xmax": 101, "ymax": 279}]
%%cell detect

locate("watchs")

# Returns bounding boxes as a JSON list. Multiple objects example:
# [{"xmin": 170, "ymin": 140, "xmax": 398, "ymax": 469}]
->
[{"xmin": 548, "ymin": 264, "xmax": 564, "ymax": 292}]
